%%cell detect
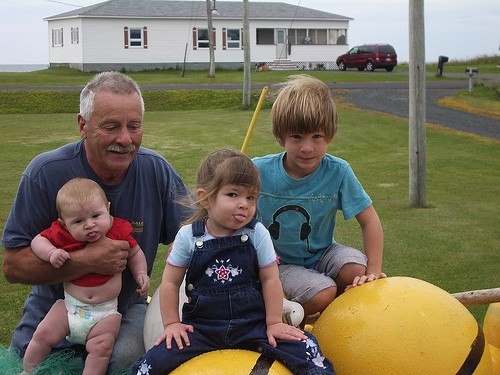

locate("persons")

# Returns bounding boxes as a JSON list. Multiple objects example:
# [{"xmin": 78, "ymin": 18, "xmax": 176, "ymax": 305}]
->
[
  {"xmin": 21, "ymin": 178, "xmax": 150, "ymax": 375},
  {"xmin": 251, "ymin": 74, "xmax": 387, "ymax": 331},
  {"xmin": 1, "ymin": 71, "xmax": 202, "ymax": 375},
  {"xmin": 136, "ymin": 148, "xmax": 338, "ymax": 375}
]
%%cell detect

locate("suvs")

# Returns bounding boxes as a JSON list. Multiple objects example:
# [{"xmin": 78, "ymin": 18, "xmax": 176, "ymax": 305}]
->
[{"xmin": 336, "ymin": 43, "xmax": 397, "ymax": 72}]
